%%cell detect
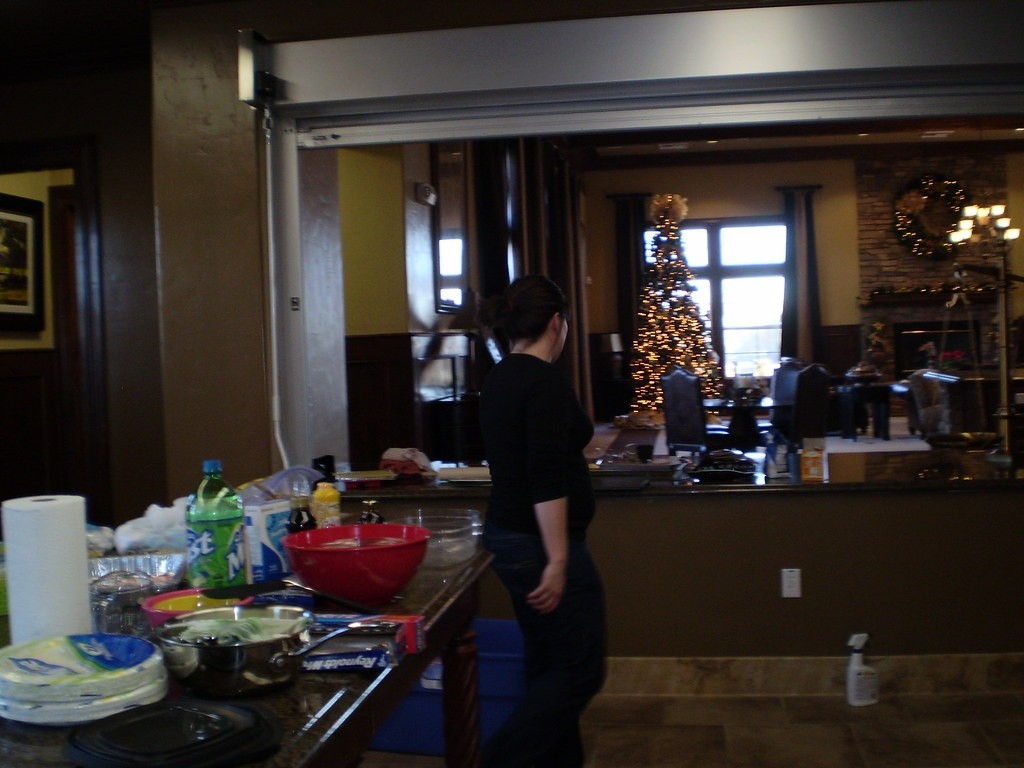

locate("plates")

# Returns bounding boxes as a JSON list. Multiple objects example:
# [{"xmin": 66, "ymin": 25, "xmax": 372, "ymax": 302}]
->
[
  {"xmin": 0, "ymin": 632, "xmax": 169, "ymax": 726},
  {"xmin": 66, "ymin": 699, "xmax": 274, "ymax": 768}
]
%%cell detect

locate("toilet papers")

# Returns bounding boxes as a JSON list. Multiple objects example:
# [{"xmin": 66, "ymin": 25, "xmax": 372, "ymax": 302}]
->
[{"xmin": 1, "ymin": 492, "xmax": 93, "ymax": 644}]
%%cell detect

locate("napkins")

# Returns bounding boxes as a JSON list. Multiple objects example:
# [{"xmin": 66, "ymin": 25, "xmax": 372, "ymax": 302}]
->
[{"xmin": 2, "ymin": 495, "xmax": 91, "ymax": 644}]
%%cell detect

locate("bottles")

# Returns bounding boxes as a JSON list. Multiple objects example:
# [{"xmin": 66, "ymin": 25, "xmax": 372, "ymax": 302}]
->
[
  {"xmin": 183, "ymin": 459, "xmax": 248, "ymax": 587},
  {"xmin": 358, "ymin": 499, "xmax": 386, "ymax": 523},
  {"xmin": 285, "ymin": 496, "xmax": 317, "ymax": 533},
  {"xmin": 309, "ymin": 482, "xmax": 341, "ymax": 528}
]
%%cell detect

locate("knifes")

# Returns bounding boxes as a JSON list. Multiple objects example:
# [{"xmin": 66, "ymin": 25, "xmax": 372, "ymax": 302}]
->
[{"xmin": 198, "ymin": 579, "xmax": 287, "ymax": 600}]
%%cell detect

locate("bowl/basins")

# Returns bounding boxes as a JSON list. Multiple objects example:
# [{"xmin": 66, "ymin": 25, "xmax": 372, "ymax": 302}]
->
[
  {"xmin": 141, "ymin": 587, "xmax": 255, "ymax": 633},
  {"xmin": 278, "ymin": 522, "xmax": 432, "ymax": 612},
  {"xmin": 395, "ymin": 508, "xmax": 485, "ymax": 569}
]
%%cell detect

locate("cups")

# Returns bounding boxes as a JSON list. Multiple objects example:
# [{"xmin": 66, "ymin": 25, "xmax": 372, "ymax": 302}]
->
[{"xmin": 87, "ymin": 576, "xmax": 158, "ymax": 643}]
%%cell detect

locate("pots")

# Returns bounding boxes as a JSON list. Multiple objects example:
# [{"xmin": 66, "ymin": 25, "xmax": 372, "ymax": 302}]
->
[{"xmin": 153, "ymin": 605, "xmax": 400, "ymax": 700}]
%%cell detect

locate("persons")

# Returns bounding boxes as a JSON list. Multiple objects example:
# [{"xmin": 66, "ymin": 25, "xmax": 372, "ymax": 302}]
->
[{"xmin": 477, "ymin": 274, "xmax": 608, "ymax": 768}]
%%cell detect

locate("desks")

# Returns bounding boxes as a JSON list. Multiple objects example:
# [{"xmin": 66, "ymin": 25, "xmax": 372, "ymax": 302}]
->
[
  {"xmin": 705, "ymin": 397, "xmax": 792, "ymax": 433},
  {"xmin": 0, "ymin": 511, "xmax": 494, "ymax": 768}
]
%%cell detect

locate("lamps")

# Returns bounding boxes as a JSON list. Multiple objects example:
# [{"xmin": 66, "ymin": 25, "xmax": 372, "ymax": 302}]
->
[{"xmin": 237, "ymin": 27, "xmax": 276, "ymax": 110}]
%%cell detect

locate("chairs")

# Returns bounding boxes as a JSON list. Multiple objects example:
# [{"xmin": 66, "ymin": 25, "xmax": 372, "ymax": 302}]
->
[
  {"xmin": 659, "ymin": 370, "xmax": 731, "ymax": 457},
  {"xmin": 772, "ymin": 357, "xmax": 802, "ymax": 445},
  {"xmin": 664, "ymin": 363, "xmax": 729, "ymax": 430},
  {"xmin": 790, "ymin": 362, "xmax": 831, "ymax": 448}
]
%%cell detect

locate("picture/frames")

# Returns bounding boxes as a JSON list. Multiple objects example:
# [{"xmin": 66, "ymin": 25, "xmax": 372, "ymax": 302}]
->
[{"xmin": 0, "ymin": 192, "xmax": 45, "ymax": 333}]
